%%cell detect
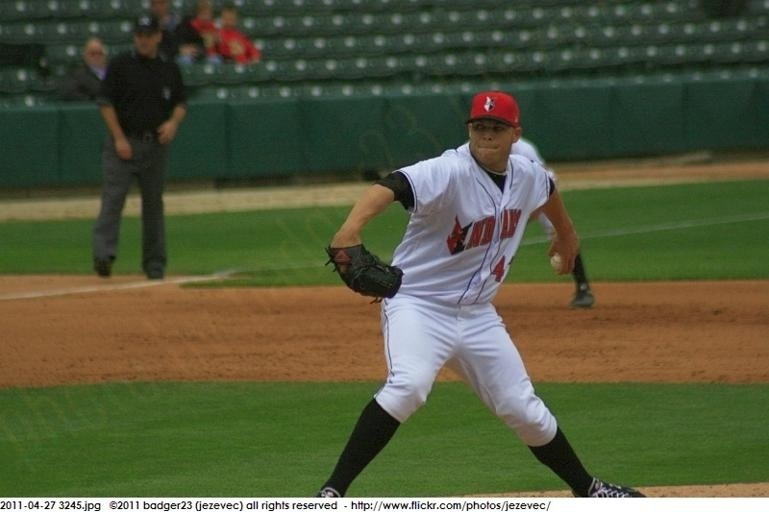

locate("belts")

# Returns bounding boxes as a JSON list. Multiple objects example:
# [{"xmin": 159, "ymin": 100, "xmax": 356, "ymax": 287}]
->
[{"xmin": 139, "ymin": 134, "xmax": 157, "ymax": 143}]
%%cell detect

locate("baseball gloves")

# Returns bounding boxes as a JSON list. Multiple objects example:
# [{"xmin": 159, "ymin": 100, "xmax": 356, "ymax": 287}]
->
[{"xmin": 325, "ymin": 244, "xmax": 404, "ymax": 298}]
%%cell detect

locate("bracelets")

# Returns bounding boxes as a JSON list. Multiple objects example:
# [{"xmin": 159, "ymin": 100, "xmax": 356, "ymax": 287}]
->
[{"xmin": 167, "ymin": 116, "xmax": 184, "ymax": 127}]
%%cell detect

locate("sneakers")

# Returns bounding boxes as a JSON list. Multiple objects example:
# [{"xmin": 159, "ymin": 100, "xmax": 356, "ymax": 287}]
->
[
  {"xmin": 141, "ymin": 258, "xmax": 164, "ymax": 278},
  {"xmin": 94, "ymin": 257, "xmax": 113, "ymax": 276},
  {"xmin": 571, "ymin": 289, "xmax": 594, "ymax": 306},
  {"xmin": 587, "ymin": 475, "xmax": 644, "ymax": 497}
]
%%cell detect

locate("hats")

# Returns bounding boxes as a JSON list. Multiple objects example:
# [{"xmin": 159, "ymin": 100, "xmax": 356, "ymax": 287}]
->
[
  {"xmin": 466, "ymin": 90, "xmax": 520, "ymax": 127},
  {"xmin": 134, "ymin": 9, "xmax": 159, "ymax": 34}
]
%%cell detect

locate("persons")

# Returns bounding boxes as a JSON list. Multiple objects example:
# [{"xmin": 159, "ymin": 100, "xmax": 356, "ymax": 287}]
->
[
  {"xmin": 315, "ymin": 90, "xmax": 645, "ymax": 498},
  {"xmin": 67, "ymin": 39, "xmax": 115, "ymax": 102},
  {"xmin": 90, "ymin": 13, "xmax": 188, "ymax": 279},
  {"xmin": 151, "ymin": 1, "xmax": 259, "ymax": 67}
]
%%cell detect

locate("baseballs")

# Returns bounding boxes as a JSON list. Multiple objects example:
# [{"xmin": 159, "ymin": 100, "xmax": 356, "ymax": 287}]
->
[{"xmin": 550, "ymin": 253, "xmax": 563, "ymax": 270}]
